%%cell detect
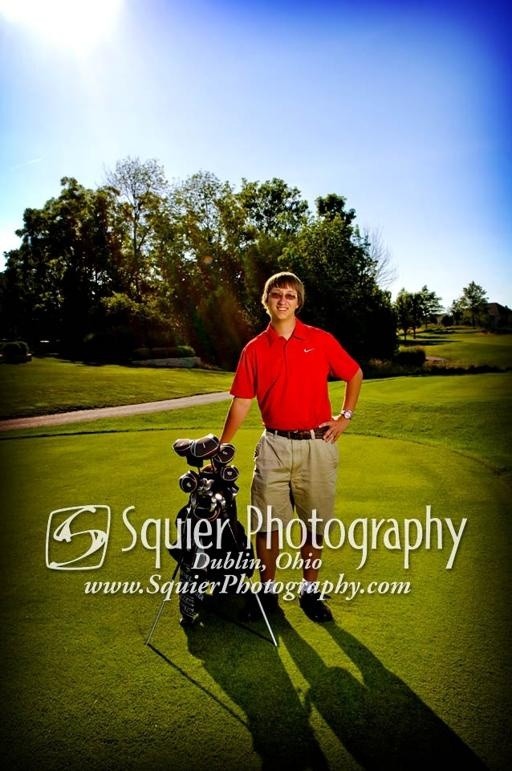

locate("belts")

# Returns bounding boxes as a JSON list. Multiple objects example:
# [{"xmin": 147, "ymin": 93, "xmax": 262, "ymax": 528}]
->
[{"xmin": 266, "ymin": 427, "xmax": 330, "ymax": 441}]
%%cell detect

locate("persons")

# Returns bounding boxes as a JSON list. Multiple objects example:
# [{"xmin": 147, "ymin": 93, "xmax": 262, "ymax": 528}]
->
[{"xmin": 219, "ymin": 271, "xmax": 364, "ymax": 625}]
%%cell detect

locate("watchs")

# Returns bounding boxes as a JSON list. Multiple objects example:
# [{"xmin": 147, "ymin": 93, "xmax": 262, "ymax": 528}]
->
[{"xmin": 340, "ymin": 410, "xmax": 353, "ymax": 421}]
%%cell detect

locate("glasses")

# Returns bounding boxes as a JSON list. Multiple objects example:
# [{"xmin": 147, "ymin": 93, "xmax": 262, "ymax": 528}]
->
[{"xmin": 269, "ymin": 291, "xmax": 296, "ymax": 300}]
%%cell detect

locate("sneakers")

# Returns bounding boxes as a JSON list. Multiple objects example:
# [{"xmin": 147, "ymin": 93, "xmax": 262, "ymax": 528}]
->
[
  {"xmin": 255, "ymin": 584, "xmax": 285, "ymax": 625},
  {"xmin": 298, "ymin": 587, "xmax": 333, "ymax": 625}
]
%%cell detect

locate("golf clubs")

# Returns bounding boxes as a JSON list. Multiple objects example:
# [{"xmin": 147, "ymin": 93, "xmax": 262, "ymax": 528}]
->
[{"xmin": 144, "ymin": 434, "xmax": 278, "ymax": 647}]
